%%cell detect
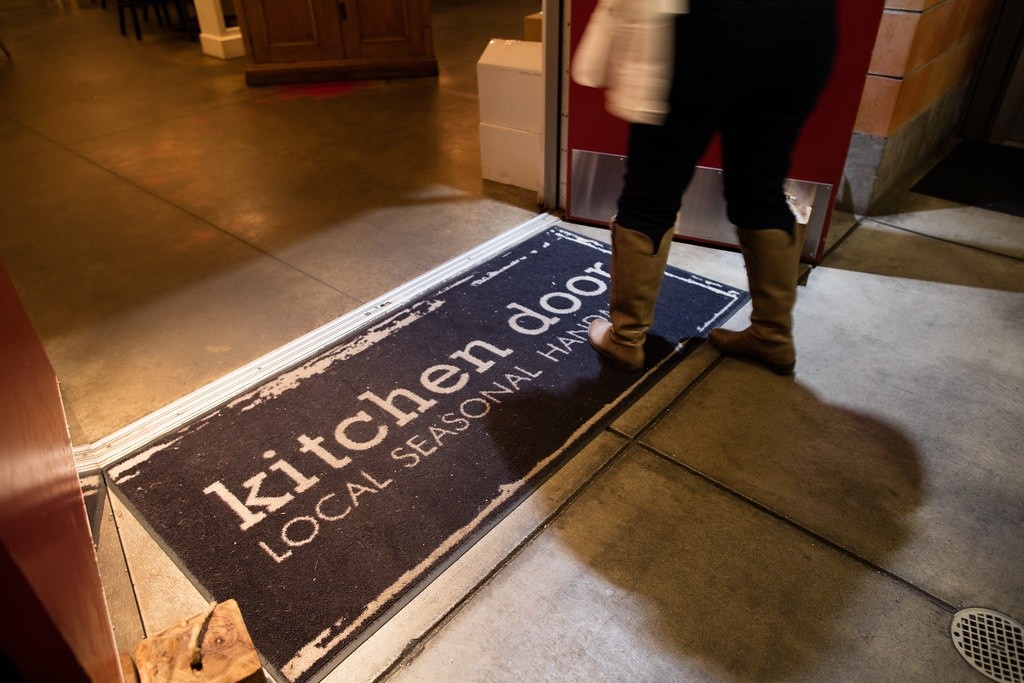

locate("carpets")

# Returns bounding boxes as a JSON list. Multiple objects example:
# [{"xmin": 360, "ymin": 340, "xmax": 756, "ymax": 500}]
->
[{"xmin": 99, "ymin": 225, "xmax": 750, "ymax": 683}]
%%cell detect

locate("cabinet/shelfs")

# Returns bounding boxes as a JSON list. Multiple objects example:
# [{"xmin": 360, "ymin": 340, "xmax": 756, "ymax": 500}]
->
[{"xmin": 233, "ymin": 0, "xmax": 440, "ymax": 88}]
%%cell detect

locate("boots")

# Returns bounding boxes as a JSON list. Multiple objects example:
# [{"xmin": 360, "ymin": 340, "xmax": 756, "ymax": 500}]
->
[
  {"xmin": 708, "ymin": 219, "xmax": 800, "ymax": 377},
  {"xmin": 587, "ymin": 215, "xmax": 675, "ymax": 368}
]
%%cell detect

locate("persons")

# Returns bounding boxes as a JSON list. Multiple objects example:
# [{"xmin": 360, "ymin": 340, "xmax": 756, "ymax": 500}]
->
[{"xmin": 589, "ymin": 1, "xmax": 839, "ymax": 374}]
